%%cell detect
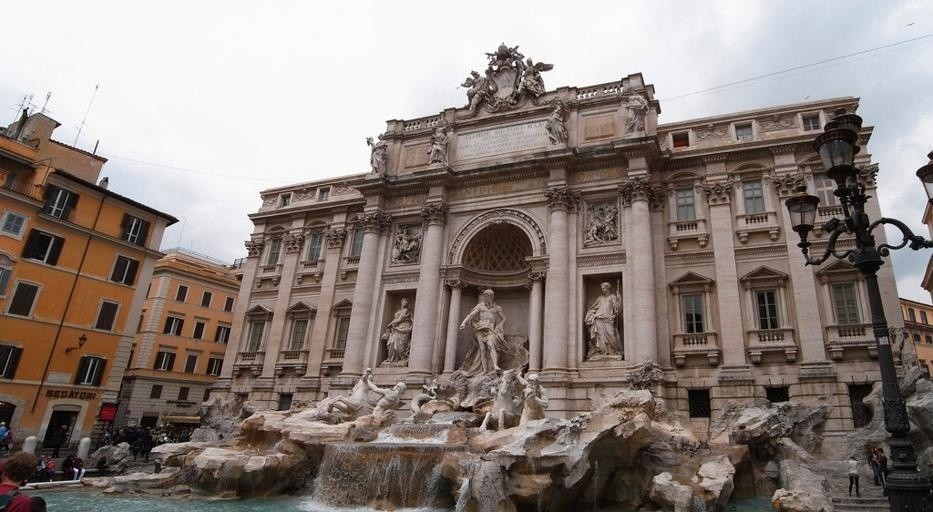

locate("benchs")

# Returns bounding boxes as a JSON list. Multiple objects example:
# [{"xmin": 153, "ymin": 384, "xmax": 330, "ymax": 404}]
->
[{"xmin": 52, "ymin": 458, "xmax": 100, "ymax": 480}]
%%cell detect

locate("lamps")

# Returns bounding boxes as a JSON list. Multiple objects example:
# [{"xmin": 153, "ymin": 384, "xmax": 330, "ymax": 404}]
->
[{"xmin": 65, "ymin": 334, "xmax": 87, "ymax": 353}]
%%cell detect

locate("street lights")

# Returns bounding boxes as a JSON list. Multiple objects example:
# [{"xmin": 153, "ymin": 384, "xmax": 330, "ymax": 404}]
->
[{"xmin": 779, "ymin": 103, "xmax": 931, "ymax": 510}]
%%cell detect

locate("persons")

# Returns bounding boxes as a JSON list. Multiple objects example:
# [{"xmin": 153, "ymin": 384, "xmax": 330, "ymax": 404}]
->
[
  {"xmin": 457, "ymin": 288, "xmax": 506, "ymax": 376},
  {"xmin": 867, "ymin": 446, "xmax": 881, "ymax": 487},
  {"xmin": 584, "ymin": 205, "xmax": 603, "ymax": 242},
  {"xmin": 0, "ymin": 450, "xmax": 46, "ymax": 512},
  {"xmin": 515, "ymin": 57, "xmax": 542, "ymax": 99},
  {"xmin": 426, "ymin": 118, "xmax": 450, "ymax": 164},
  {"xmin": 0, "ymin": 420, "xmax": 197, "ymax": 481},
  {"xmin": 617, "ymin": 87, "xmax": 647, "ymax": 132},
  {"xmin": 368, "ymin": 134, "xmax": 388, "ymax": 174},
  {"xmin": 586, "ymin": 282, "xmax": 624, "ymax": 356},
  {"xmin": 383, "ymin": 297, "xmax": 412, "ymax": 367},
  {"xmin": 603, "ymin": 206, "xmax": 617, "ymax": 241},
  {"xmin": 546, "ymin": 100, "xmax": 569, "ymax": 143},
  {"xmin": 844, "ymin": 452, "xmax": 862, "ymax": 498},
  {"xmin": 394, "ymin": 226, "xmax": 420, "ymax": 262},
  {"xmin": 467, "ymin": 69, "xmax": 489, "ymax": 110},
  {"xmin": 873, "ymin": 447, "xmax": 887, "ymax": 497},
  {"xmin": 318, "ymin": 367, "xmax": 552, "ymax": 443}
]
